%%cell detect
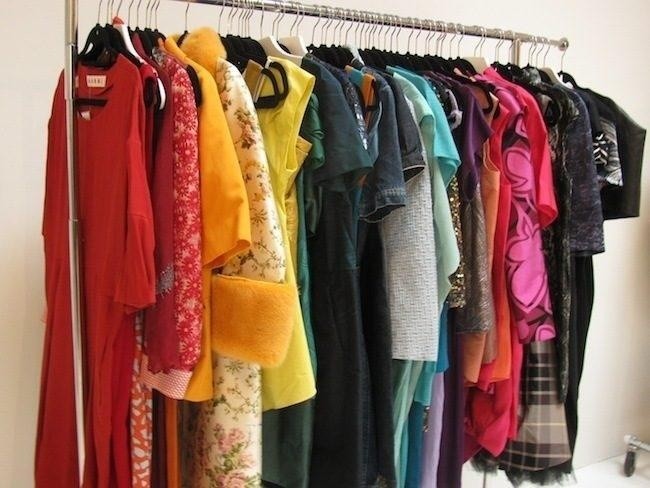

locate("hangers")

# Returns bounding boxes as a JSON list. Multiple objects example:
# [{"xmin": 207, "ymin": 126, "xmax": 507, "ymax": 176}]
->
[{"xmin": 74, "ymin": 0, "xmax": 602, "ymax": 112}]
